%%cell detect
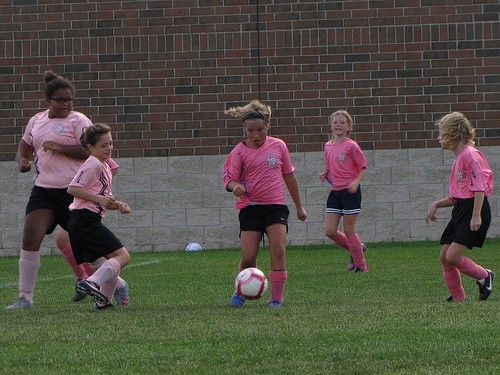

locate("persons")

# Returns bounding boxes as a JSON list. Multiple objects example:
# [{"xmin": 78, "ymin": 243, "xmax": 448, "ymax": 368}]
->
[
  {"xmin": 67, "ymin": 123, "xmax": 131, "ymax": 309},
  {"xmin": 6, "ymin": 71, "xmax": 95, "ymax": 310},
  {"xmin": 426, "ymin": 112, "xmax": 495, "ymax": 302},
  {"xmin": 222, "ymin": 100, "xmax": 307, "ymax": 308},
  {"xmin": 318, "ymin": 110, "xmax": 367, "ymax": 273},
  {"xmin": 56, "ymin": 158, "xmax": 119, "ymax": 301}
]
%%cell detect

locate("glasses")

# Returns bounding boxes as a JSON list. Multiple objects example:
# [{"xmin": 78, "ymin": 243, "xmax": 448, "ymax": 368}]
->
[{"xmin": 50, "ymin": 97, "xmax": 74, "ymax": 104}]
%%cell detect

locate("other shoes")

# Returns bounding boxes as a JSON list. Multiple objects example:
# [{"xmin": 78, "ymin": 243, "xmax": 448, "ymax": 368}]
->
[
  {"xmin": 71, "ymin": 277, "xmax": 88, "ymax": 302},
  {"xmin": 6, "ymin": 294, "xmax": 34, "ymax": 309},
  {"xmin": 352, "ymin": 265, "xmax": 369, "ymax": 273},
  {"xmin": 114, "ymin": 280, "xmax": 130, "ymax": 307},
  {"xmin": 348, "ymin": 243, "xmax": 366, "ymax": 270},
  {"xmin": 231, "ymin": 290, "xmax": 246, "ymax": 307},
  {"xmin": 266, "ymin": 300, "xmax": 281, "ymax": 307}
]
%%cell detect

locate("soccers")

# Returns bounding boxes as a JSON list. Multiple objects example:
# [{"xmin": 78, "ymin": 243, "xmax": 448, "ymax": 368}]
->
[
  {"xmin": 234, "ymin": 267, "xmax": 268, "ymax": 300},
  {"xmin": 184, "ymin": 242, "xmax": 205, "ymax": 252}
]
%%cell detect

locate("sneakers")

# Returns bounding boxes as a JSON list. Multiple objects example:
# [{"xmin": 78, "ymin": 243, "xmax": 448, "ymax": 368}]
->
[
  {"xmin": 444, "ymin": 296, "xmax": 467, "ymax": 304},
  {"xmin": 476, "ymin": 269, "xmax": 495, "ymax": 300},
  {"xmin": 75, "ymin": 278, "xmax": 109, "ymax": 304},
  {"xmin": 93, "ymin": 301, "xmax": 115, "ymax": 309}
]
